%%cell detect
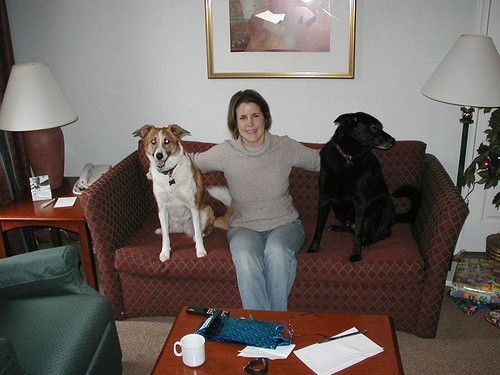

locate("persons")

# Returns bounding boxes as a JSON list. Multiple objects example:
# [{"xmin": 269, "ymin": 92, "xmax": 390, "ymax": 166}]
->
[{"xmin": 145, "ymin": 88, "xmax": 322, "ymax": 311}]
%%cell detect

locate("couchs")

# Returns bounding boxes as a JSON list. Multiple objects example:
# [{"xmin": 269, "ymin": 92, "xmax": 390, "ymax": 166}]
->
[
  {"xmin": 79, "ymin": 135, "xmax": 470, "ymax": 340},
  {"xmin": 0, "ymin": 245, "xmax": 122, "ymax": 375}
]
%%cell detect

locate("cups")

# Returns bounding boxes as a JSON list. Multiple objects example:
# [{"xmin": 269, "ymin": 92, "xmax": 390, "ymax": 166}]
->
[{"xmin": 174, "ymin": 333, "xmax": 206, "ymax": 367}]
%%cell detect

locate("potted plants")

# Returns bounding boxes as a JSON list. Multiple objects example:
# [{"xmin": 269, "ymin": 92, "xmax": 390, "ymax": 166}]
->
[{"xmin": 462, "ymin": 106, "xmax": 499, "ymax": 262}]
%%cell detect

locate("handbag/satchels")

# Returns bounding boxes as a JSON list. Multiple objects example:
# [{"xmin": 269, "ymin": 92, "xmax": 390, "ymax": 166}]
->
[{"xmin": 195, "ymin": 310, "xmax": 293, "ymax": 349}]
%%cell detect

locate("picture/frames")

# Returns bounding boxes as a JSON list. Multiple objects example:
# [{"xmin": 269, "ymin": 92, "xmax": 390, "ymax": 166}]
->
[{"xmin": 204, "ymin": 1, "xmax": 356, "ymax": 80}]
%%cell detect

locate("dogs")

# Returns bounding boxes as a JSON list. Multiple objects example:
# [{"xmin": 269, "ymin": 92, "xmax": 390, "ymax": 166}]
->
[
  {"xmin": 132, "ymin": 125, "xmax": 233, "ymax": 262},
  {"xmin": 306, "ymin": 112, "xmax": 421, "ymax": 262}
]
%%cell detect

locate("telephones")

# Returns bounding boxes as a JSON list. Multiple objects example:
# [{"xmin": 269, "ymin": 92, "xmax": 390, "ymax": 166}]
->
[{"xmin": 73, "ymin": 163, "xmax": 111, "ymax": 195}]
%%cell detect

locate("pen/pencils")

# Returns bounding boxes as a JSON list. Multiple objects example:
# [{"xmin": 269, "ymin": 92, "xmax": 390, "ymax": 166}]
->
[
  {"xmin": 318, "ymin": 329, "xmax": 367, "ymax": 344},
  {"xmin": 43, "ymin": 195, "xmax": 59, "ymax": 208}
]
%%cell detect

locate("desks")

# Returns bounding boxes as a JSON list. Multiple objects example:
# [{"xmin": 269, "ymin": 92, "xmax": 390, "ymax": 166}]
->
[
  {"xmin": 151, "ymin": 305, "xmax": 405, "ymax": 374},
  {"xmin": 0, "ymin": 176, "xmax": 100, "ymax": 291}
]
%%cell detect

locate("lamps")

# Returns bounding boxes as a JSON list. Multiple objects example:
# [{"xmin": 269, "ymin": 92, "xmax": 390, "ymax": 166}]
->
[
  {"xmin": 420, "ymin": 33, "xmax": 499, "ymax": 286},
  {"xmin": 0, "ymin": 60, "xmax": 79, "ymax": 193}
]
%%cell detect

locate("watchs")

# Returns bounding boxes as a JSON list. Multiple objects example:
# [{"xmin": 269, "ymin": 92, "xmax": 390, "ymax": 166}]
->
[{"xmin": 243, "ymin": 357, "xmax": 269, "ymax": 375}]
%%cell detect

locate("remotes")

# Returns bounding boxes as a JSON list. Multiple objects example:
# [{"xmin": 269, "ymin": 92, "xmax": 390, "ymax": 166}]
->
[{"xmin": 187, "ymin": 306, "xmax": 230, "ymax": 319}]
